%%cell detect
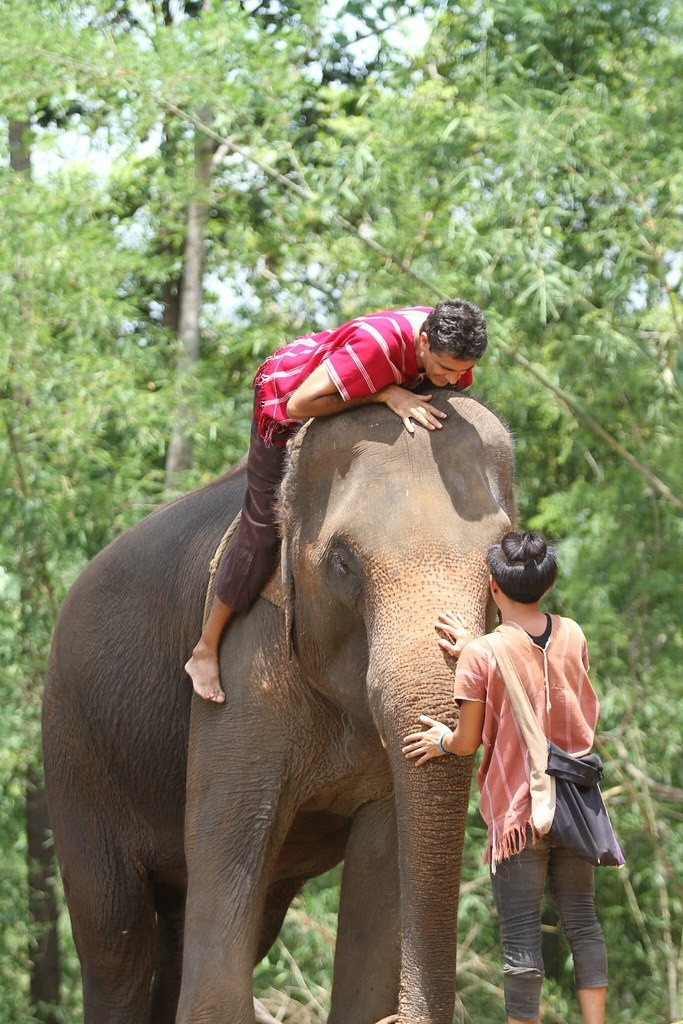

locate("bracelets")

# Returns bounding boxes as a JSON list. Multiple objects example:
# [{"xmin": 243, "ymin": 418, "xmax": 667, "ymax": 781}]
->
[{"xmin": 439, "ymin": 729, "xmax": 451, "ymax": 755}]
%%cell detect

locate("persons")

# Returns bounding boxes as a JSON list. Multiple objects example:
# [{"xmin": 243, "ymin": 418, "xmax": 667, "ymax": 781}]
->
[
  {"xmin": 183, "ymin": 296, "xmax": 488, "ymax": 702},
  {"xmin": 403, "ymin": 527, "xmax": 626, "ymax": 1024}
]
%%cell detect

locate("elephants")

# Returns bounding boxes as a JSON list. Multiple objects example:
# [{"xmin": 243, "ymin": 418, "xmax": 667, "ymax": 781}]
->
[{"xmin": 42, "ymin": 388, "xmax": 519, "ymax": 1024}]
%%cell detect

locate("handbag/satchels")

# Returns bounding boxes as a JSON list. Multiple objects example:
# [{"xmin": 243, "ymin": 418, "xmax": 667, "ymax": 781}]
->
[{"xmin": 527, "ymin": 741, "xmax": 625, "ymax": 867}]
